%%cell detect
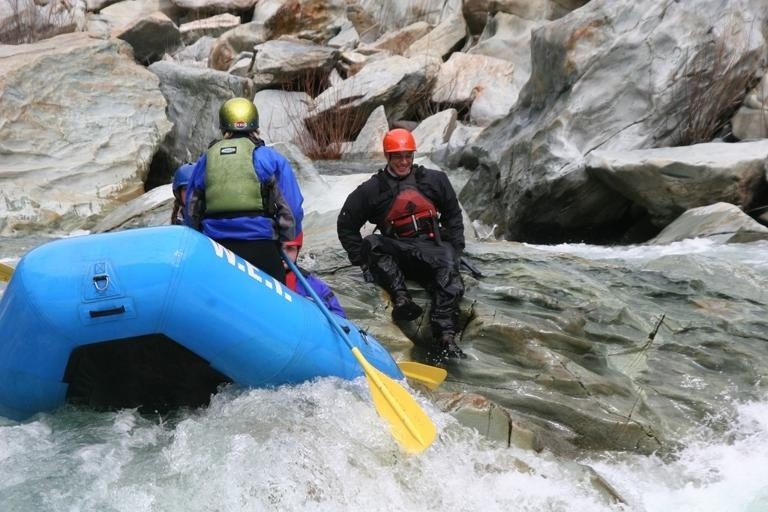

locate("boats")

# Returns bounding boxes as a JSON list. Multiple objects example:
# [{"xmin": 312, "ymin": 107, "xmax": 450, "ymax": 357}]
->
[{"xmin": 0, "ymin": 225, "xmax": 404, "ymax": 424}]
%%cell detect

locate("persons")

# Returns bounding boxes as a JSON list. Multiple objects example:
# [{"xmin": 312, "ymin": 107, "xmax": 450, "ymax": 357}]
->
[
  {"xmin": 336, "ymin": 130, "xmax": 466, "ymax": 357},
  {"xmin": 279, "ymin": 229, "xmax": 348, "ymax": 318},
  {"xmin": 189, "ymin": 96, "xmax": 302, "ymax": 278},
  {"xmin": 170, "ymin": 161, "xmax": 194, "ymax": 224}
]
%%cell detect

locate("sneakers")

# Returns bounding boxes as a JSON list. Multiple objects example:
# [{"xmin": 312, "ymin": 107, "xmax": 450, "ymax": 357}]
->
[
  {"xmin": 429, "ymin": 337, "xmax": 468, "ymax": 360},
  {"xmin": 391, "ymin": 300, "xmax": 423, "ymax": 322}
]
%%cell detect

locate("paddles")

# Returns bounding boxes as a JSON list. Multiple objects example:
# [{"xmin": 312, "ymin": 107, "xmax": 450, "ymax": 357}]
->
[
  {"xmin": 282, "ymin": 250, "xmax": 438, "ymax": 454},
  {"xmin": 396, "ymin": 362, "xmax": 447, "ymax": 390}
]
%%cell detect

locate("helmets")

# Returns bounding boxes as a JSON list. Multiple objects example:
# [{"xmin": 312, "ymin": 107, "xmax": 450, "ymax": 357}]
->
[
  {"xmin": 280, "ymin": 229, "xmax": 304, "ymax": 247},
  {"xmin": 172, "ymin": 162, "xmax": 198, "ymax": 192},
  {"xmin": 219, "ymin": 96, "xmax": 259, "ymax": 132},
  {"xmin": 382, "ymin": 127, "xmax": 416, "ymax": 153}
]
